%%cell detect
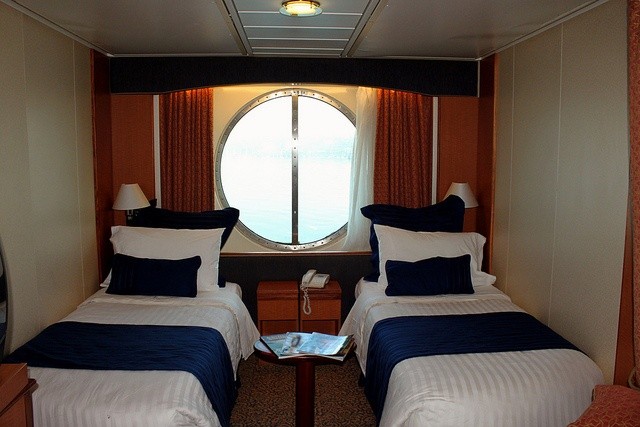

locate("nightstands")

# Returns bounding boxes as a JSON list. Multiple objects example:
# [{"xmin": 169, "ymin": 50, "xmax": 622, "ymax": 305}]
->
[
  {"xmin": 300, "ymin": 280, "xmax": 342, "ymax": 335},
  {"xmin": 256, "ymin": 279, "xmax": 300, "ymax": 336}
]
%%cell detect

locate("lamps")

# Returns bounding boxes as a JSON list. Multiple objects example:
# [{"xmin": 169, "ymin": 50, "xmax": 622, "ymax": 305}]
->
[
  {"xmin": 112, "ymin": 183, "xmax": 151, "ymax": 226},
  {"xmin": 277, "ymin": 1, "xmax": 322, "ymax": 17},
  {"xmin": 442, "ymin": 182, "xmax": 479, "ymax": 208}
]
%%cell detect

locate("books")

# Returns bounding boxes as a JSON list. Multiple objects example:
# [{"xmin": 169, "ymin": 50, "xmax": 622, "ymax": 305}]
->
[
  {"xmin": 297, "ymin": 331, "xmax": 355, "ymax": 362},
  {"xmin": 260, "ymin": 333, "xmax": 299, "ymax": 360},
  {"xmin": 280, "ymin": 331, "xmax": 312, "ymax": 357}
]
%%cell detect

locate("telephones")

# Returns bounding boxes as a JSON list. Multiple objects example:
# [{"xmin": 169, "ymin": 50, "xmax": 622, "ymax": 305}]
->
[{"xmin": 300, "ymin": 269, "xmax": 331, "ymax": 316}]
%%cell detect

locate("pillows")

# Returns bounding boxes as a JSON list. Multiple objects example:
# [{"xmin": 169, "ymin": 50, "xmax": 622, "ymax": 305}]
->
[
  {"xmin": 373, "ymin": 224, "xmax": 496, "ymax": 298},
  {"xmin": 125, "ymin": 206, "xmax": 240, "ymax": 291},
  {"xmin": 94, "ymin": 225, "xmax": 227, "ymax": 292},
  {"xmin": 103, "ymin": 252, "xmax": 201, "ymax": 298},
  {"xmin": 384, "ymin": 254, "xmax": 475, "ymax": 297},
  {"xmin": 360, "ymin": 192, "xmax": 466, "ymax": 283}
]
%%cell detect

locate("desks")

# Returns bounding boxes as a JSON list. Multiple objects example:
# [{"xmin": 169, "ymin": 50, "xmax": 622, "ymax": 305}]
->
[{"xmin": 254, "ymin": 339, "xmax": 358, "ymax": 426}]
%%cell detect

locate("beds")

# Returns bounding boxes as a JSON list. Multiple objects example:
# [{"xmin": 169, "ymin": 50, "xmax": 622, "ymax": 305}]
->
[
  {"xmin": 337, "ymin": 277, "xmax": 606, "ymax": 427},
  {"xmin": 0, "ymin": 281, "xmax": 261, "ymax": 426}
]
